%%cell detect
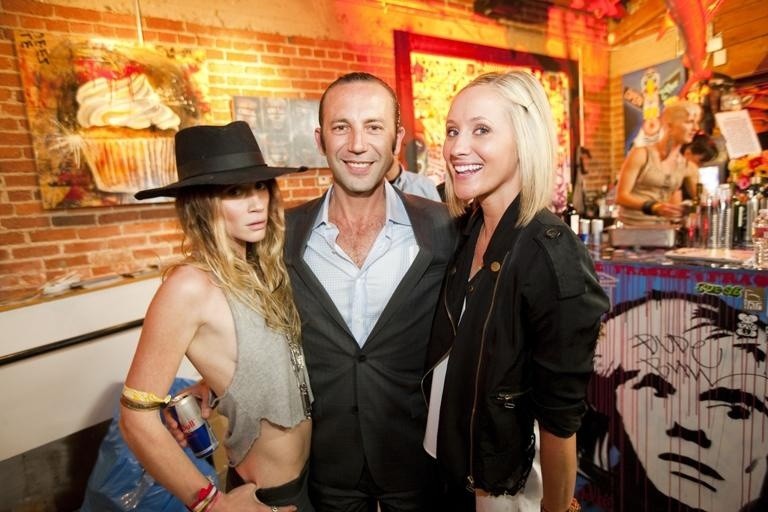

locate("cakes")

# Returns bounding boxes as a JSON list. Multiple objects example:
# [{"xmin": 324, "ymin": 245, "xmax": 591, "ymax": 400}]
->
[{"xmin": 76, "ymin": 67, "xmax": 183, "ymax": 195}]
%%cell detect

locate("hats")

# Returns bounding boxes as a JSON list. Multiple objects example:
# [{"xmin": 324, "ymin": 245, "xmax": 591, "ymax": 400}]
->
[{"xmin": 134, "ymin": 121, "xmax": 308, "ymax": 200}]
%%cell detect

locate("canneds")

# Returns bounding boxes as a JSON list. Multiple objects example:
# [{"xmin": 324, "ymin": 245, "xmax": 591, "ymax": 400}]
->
[{"xmin": 167, "ymin": 391, "xmax": 219, "ymax": 458}]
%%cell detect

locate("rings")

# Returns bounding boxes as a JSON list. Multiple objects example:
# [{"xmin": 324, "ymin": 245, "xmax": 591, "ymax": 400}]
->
[{"xmin": 271, "ymin": 507, "xmax": 278, "ymax": 512}]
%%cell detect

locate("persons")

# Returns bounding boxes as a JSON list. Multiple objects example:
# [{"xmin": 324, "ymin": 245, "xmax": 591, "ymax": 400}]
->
[
  {"xmin": 610, "ymin": 98, "xmax": 716, "ymax": 247},
  {"xmin": 115, "ymin": 117, "xmax": 318, "ymax": 512},
  {"xmin": 159, "ymin": 70, "xmax": 474, "ymax": 511},
  {"xmin": 383, "ymin": 154, "xmax": 442, "ymax": 204},
  {"xmin": 404, "ymin": 67, "xmax": 612, "ymax": 512},
  {"xmin": 668, "ymin": 132, "xmax": 720, "ymax": 206}
]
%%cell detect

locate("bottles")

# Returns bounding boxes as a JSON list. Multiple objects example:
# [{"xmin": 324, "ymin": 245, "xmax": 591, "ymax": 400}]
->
[
  {"xmin": 750, "ymin": 209, "xmax": 768, "ymax": 273},
  {"xmin": 687, "ymin": 182, "xmax": 760, "ymax": 249},
  {"xmin": 565, "ymin": 192, "xmax": 580, "ymax": 238}
]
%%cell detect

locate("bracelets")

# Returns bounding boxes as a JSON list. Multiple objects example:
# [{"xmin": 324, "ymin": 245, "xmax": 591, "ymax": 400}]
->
[
  {"xmin": 651, "ymin": 202, "xmax": 664, "ymax": 213},
  {"xmin": 188, "ymin": 484, "xmax": 221, "ymax": 512},
  {"xmin": 536, "ymin": 496, "xmax": 582, "ymax": 512},
  {"xmin": 641, "ymin": 199, "xmax": 655, "ymax": 215}
]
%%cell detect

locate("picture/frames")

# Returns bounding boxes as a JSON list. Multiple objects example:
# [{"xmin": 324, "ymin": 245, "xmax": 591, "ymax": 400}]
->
[{"xmin": 392, "ymin": 27, "xmax": 580, "ymax": 224}]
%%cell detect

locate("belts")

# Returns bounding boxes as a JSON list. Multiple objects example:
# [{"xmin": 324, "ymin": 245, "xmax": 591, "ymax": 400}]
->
[{"xmin": 229, "ymin": 461, "xmax": 310, "ymax": 502}]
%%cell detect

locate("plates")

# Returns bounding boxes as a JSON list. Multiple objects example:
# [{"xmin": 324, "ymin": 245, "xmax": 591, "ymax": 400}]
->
[{"xmin": 666, "ymin": 246, "xmax": 754, "ymax": 268}]
